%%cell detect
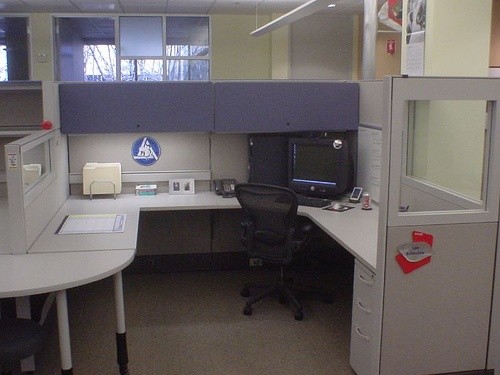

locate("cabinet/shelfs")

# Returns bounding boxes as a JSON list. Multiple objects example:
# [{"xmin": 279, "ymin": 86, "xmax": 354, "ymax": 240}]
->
[{"xmin": 349, "ymin": 259, "xmax": 379, "ymax": 375}]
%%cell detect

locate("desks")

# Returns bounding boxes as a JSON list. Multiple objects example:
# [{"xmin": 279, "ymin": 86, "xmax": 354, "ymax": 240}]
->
[{"xmin": 0, "ymin": 191, "xmax": 380, "ymax": 375}]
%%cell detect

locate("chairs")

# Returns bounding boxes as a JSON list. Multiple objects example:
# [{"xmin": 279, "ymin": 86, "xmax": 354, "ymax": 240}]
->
[{"xmin": 235, "ymin": 183, "xmax": 313, "ymax": 320}]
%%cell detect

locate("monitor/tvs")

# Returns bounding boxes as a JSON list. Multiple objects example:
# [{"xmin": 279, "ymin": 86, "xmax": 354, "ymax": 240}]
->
[{"xmin": 284, "ymin": 137, "xmax": 350, "ymax": 200}]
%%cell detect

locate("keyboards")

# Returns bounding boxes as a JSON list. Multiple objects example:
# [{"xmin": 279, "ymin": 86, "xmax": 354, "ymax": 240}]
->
[{"xmin": 295, "ymin": 195, "xmax": 331, "ymax": 208}]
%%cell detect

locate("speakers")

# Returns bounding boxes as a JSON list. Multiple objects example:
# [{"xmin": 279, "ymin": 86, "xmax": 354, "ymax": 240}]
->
[{"xmin": 248, "ymin": 136, "xmax": 289, "ymax": 187}]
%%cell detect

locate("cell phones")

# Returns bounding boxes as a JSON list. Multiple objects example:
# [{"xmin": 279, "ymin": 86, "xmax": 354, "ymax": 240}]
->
[{"xmin": 350, "ymin": 187, "xmax": 362, "ymax": 201}]
